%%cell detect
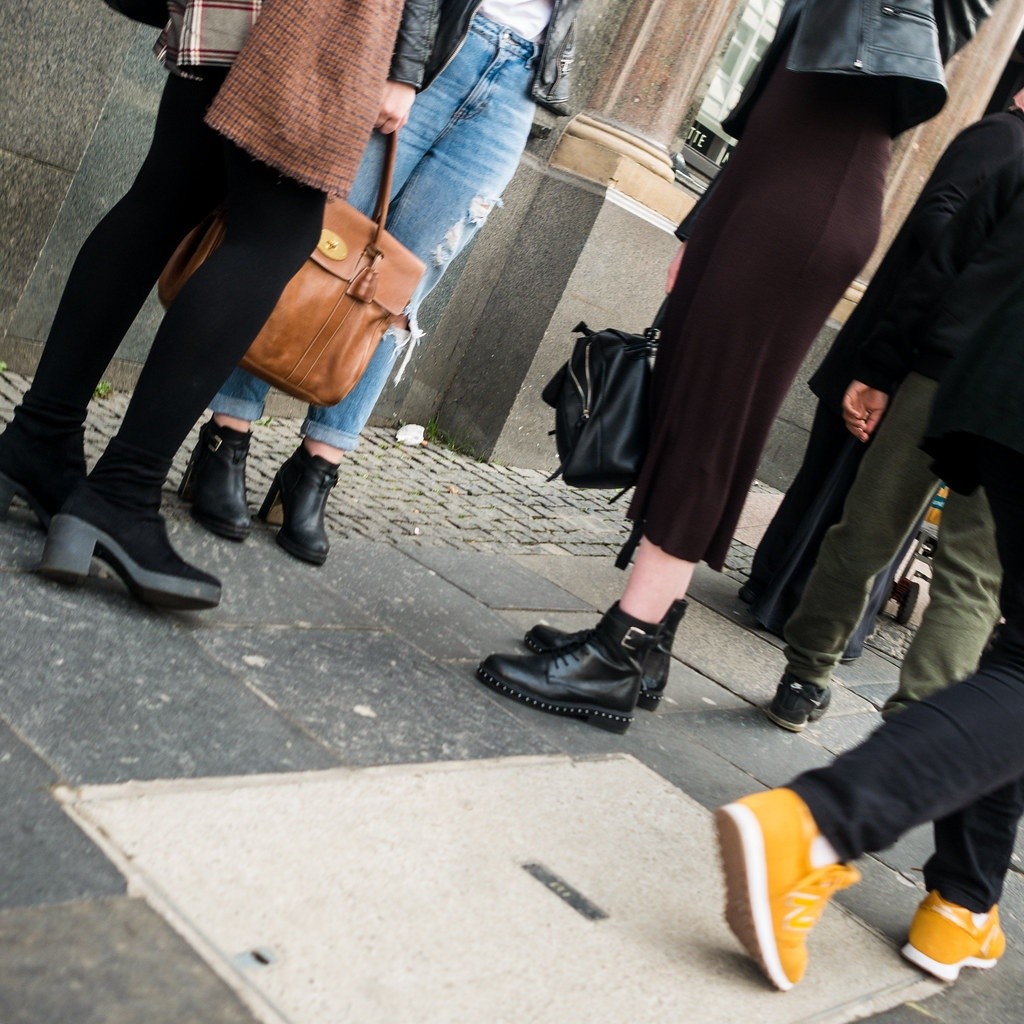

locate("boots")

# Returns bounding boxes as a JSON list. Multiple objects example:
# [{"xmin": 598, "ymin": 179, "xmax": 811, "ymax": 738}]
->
[
  {"xmin": 525, "ymin": 598, "xmax": 687, "ymax": 712},
  {"xmin": 475, "ymin": 601, "xmax": 666, "ymax": 733}
]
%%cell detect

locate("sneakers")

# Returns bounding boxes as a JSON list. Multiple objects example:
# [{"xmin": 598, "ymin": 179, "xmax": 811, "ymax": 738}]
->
[
  {"xmin": 766, "ymin": 674, "xmax": 831, "ymax": 733},
  {"xmin": 881, "ymin": 692, "xmax": 911, "ymax": 719},
  {"xmin": 715, "ymin": 787, "xmax": 864, "ymax": 992},
  {"xmin": 901, "ymin": 886, "xmax": 1007, "ymax": 983}
]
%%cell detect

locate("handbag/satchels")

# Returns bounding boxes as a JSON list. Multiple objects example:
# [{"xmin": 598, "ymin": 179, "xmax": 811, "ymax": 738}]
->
[
  {"xmin": 543, "ymin": 320, "xmax": 667, "ymax": 488},
  {"xmin": 155, "ymin": 119, "xmax": 423, "ymax": 408}
]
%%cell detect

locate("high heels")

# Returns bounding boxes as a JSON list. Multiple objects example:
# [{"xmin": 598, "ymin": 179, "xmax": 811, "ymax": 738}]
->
[
  {"xmin": 257, "ymin": 438, "xmax": 340, "ymax": 565},
  {"xmin": 0, "ymin": 402, "xmax": 87, "ymax": 522},
  {"xmin": 178, "ymin": 412, "xmax": 253, "ymax": 541},
  {"xmin": 37, "ymin": 455, "xmax": 222, "ymax": 610}
]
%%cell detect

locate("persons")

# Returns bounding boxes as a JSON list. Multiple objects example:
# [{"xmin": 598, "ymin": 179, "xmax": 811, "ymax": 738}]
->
[
  {"xmin": 476, "ymin": 0, "xmax": 1024, "ymax": 989},
  {"xmin": 175, "ymin": 0, "xmax": 582, "ymax": 563},
  {"xmin": 0, "ymin": 0, "xmax": 408, "ymax": 611}
]
